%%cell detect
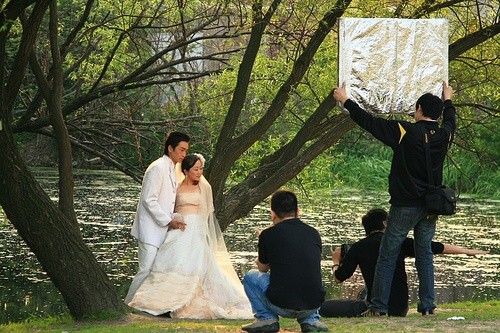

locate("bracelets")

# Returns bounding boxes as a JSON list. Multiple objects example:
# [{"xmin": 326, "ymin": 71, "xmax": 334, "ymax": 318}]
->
[{"xmin": 332, "ymin": 263, "xmax": 340, "ymax": 270}]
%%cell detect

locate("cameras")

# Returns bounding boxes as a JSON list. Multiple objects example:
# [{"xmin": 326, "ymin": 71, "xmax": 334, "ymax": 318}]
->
[{"xmin": 341, "ymin": 244, "xmax": 351, "ymax": 258}]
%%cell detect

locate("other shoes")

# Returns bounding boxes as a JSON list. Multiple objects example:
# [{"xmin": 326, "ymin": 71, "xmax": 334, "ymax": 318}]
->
[
  {"xmin": 300, "ymin": 320, "xmax": 328, "ymax": 332},
  {"xmin": 356, "ymin": 308, "xmax": 388, "ymax": 317},
  {"xmin": 422, "ymin": 308, "xmax": 435, "ymax": 317},
  {"xmin": 242, "ymin": 318, "xmax": 279, "ymax": 332}
]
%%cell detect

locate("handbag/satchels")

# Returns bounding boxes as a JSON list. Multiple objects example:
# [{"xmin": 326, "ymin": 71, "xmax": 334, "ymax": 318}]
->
[{"xmin": 422, "ymin": 185, "xmax": 456, "ymax": 215}]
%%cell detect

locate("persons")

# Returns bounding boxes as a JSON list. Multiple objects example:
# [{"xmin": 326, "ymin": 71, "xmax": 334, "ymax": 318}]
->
[
  {"xmin": 334, "ymin": 81, "xmax": 457, "ymax": 317},
  {"xmin": 124, "ymin": 131, "xmax": 190, "ymax": 305},
  {"xmin": 318, "ymin": 208, "xmax": 491, "ymax": 317},
  {"xmin": 128, "ymin": 153, "xmax": 255, "ymax": 320},
  {"xmin": 242, "ymin": 190, "xmax": 329, "ymax": 333}
]
